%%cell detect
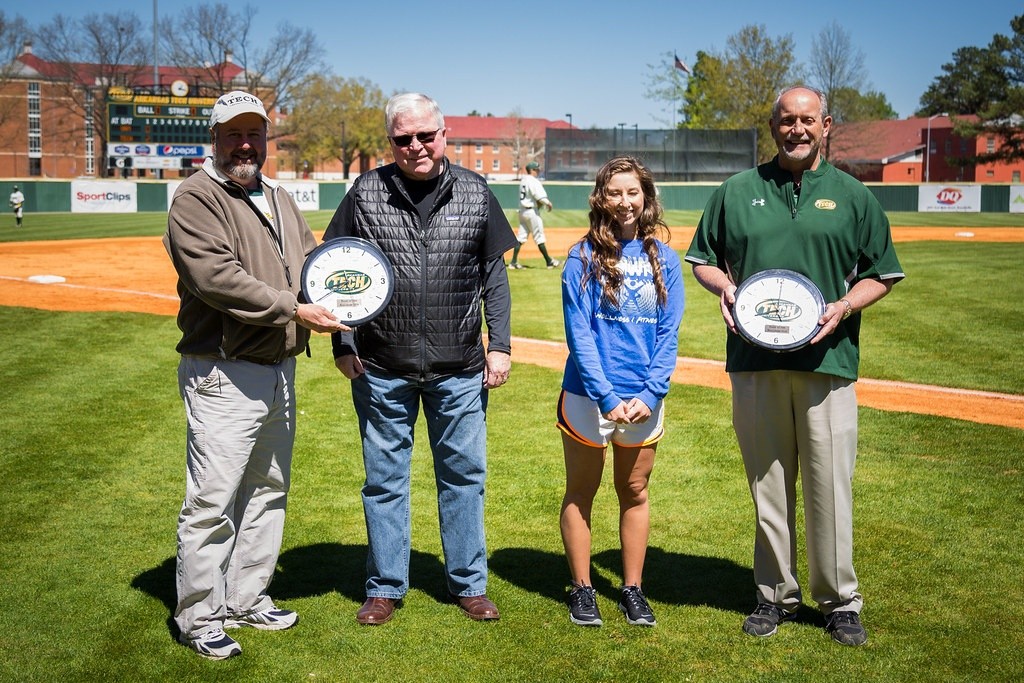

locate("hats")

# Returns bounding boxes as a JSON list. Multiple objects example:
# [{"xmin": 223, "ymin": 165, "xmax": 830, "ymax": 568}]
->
[
  {"xmin": 525, "ymin": 162, "xmax": 540, "ymax": 171},
  {"xmin": 210, "ymin": 90, "xmax": 272, "ymax": 129}
]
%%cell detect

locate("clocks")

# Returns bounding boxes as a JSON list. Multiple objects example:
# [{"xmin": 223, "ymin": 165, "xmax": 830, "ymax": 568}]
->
[
  {"xmin": 731, "ymin": 269, "xmax": 825, "ymax": 352},
  {"xmin": 170, "ymin": 79, "xmax": 188, "ymax": 96},
  {"xmin": 301, "ymin": 237, "xmax": 395, "ymax": 327}
]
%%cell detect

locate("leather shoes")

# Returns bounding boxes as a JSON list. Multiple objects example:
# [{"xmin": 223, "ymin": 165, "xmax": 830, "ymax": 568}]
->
[
  {"xmin": 445, "ymin": 585, "xmax": 499, "ymax": 619},
  {"xmin": 356, "ymin": 595, "xmax": 396, "ymax": 625}
]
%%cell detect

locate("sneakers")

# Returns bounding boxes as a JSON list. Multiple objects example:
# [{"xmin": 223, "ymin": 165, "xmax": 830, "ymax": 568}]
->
[
  {"xmin": 222, "ymin": 605, "xmax": 297, "ymax": 630},
  {"xmin": 743, "ymin": 603, "xmax": 796, "ymax": 636},
  {"xmin": 508, "ymin": 264, "xmax": 526, "ymax": 270},
  {"xmin": 620, "ymin": 586, "xmax": 656, "ymax": 625},
  {"xmin": 547, "ymin": 259, "xmax": 565, "ymax": 269},
  {"xmin": 180, "ymin": 631, "xmax": 243, "ymax": 660},
  {"xmin": 569, "ymin": 580, "xmax": 602, "ymax": 625},
  {"xmin": 825, "ymin": 610, "xmax": 865, "ymax": 645}
]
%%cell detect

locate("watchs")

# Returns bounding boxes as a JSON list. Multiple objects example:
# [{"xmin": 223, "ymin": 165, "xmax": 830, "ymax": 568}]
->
[{"xmin": 840, "ymin": 299, "xmax": 852, "ymax": 320}]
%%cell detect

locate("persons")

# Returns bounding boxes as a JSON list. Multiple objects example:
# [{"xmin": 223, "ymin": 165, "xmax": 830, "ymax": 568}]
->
[
  {"xmin": 685, "ymin": 84, "xmax": 906, "ymax": 646},
  {"xmin": 322, "ymin": 93, "xmax": 518, "ymax": 625},
  {"xmin": 162, "ymin": 90, "xmax": 351, "ymax": 660},
  {"xmin": 555, "ymin": 155, "xmax": 684, "ymax": 628},
  {"xmin": 508, "ymin": 161, "xmax": 565, "ymax": 269},
  {"xmin": 9, "ymin": 185, "xmax": 25, "ymax": 229}
]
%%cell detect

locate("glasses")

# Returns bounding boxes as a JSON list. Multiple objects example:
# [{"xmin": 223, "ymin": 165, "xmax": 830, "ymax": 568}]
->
[{"xmin": 390, "ymin": 126, "xmax": 441, "ymax": 146}]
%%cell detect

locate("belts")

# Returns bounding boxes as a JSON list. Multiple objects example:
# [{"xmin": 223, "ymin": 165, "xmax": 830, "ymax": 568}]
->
[{"xmin": 525, "ymin": 206, "xmax": 532, "ymax": 209}]
